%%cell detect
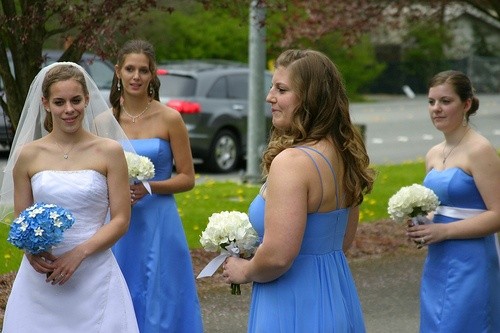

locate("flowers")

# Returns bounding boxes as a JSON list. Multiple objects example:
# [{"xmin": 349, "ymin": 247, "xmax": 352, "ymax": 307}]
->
[
  {"xmin": 387, "ymin": 183, "xmax": 441, "ymax": 249},
  {"xmin": 7, "ymin": 202, "xmax": 75, "ymax": 280},
  {"xmin": 124, "ymin": 151, "xmax": 157, "ymax": 196},
  {"xmin": 197, "ymin": 211, "xmax": 258, "ymax": 295}
]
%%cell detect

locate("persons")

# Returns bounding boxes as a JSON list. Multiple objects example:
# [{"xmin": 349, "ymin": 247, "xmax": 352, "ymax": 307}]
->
[
  {"xmin": 92, "ymin": 40, "xmax": 204, "ymax": 333},
  {"xmin": 405, "ymin": 70, "xmax": 500, "ymax": 333},
  {"xmin": 0, "ymin": 61, "xmax": 141, "ymax": 333},
  {"xmin": 222, "ymin": 49, "xmax": 376, "ymax": 333}
]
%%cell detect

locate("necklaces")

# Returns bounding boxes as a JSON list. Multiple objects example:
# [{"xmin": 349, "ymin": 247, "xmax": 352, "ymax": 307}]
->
[
  {"xmin": 443, "ymin": 127, "xmax": 472, "ymax": 163},
  {"xmin": 58, "ymin": 143, "xmax": 75, "ymax": 159},
  {"xmin": 122, "ymin": 102, "xmax": 150, "ymax": 123}
]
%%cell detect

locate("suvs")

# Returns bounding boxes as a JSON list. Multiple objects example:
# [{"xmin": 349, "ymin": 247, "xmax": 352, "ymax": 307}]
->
[
  {"xmin": 153, "ymin": 64, "xmax": 275, "ymax": 172},
  {"xmin": 0, "ymin": 50, "xmax": 118, "ymax": 128}
]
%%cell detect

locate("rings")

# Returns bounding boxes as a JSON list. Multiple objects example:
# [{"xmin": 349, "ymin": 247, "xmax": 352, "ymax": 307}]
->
[
  {"xmin": 421, "ymin": 238, "xmax": 424, "ymax": 244},
  {"xmin": 61, "ymin": 274, "xmax": 64, "ymax": 277},
  {"xmin": 223, "ymin": 265, "xmax": 225, "ymax": 270}
]
